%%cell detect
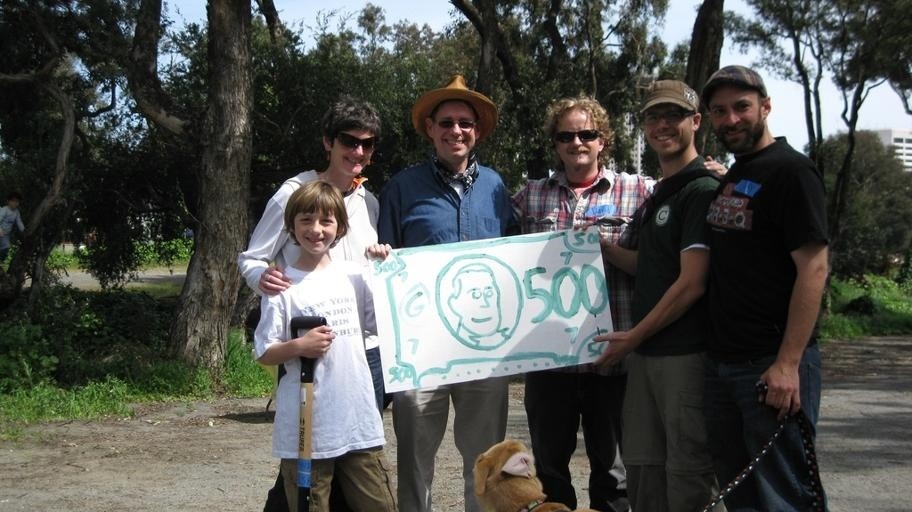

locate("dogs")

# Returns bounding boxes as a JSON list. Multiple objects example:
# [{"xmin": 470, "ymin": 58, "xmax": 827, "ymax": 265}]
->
[{"xmin": 471, "ymin": 441, "xmax": 600, "ymax": 512}]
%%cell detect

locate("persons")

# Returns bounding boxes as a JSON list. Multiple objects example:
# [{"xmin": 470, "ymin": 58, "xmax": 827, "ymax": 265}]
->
[
  {"xmin": 378, "ymin": 76, "xmax": 513, "ymax": 512},
  {"xmin": 239, "ymin": 98, "xmax": 384, "ymax": 512},
  {"xmin": 574, "ymin": 80, "xmax": 726, "ymax": 512},
  {"xmin": 254, "ymin": 179, "xmax": 396, "ymax": 512},
  {"xmin": 699, "ymin": 66, "xmax": 829, "ymax": 512},
  {"xmin": 405, "ymin": 97, "xmax": 728, "ymax": 512},
  {"xmin": 0, "ymin": 194, "xmax": 24, "ymax": 262}
]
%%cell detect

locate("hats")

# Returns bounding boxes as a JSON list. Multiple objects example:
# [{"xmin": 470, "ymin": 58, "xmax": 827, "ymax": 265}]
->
[
  {"xmin": 701, "ymin": 66, "xmax": 769, "ymax": 101},
  {"xmin": 640, "ymin": 80, "xmax": 701, "ymax": 116},
  {"xmin": 410, "ymin": 75, "xmax": 500, "ymax": 148}
]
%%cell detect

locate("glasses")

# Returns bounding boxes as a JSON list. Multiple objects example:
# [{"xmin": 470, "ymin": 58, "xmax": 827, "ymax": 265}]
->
[
  {"xmin": 434, "ymin": 118, "xmax": 478, "ymax": 129},
  {"xmin": 335, "ymin": 132, "xmax": 377, "ymax": 151},
  {"xmin": 554, "ymin": 129, "xmax": 604, "ymax": 145}
]
